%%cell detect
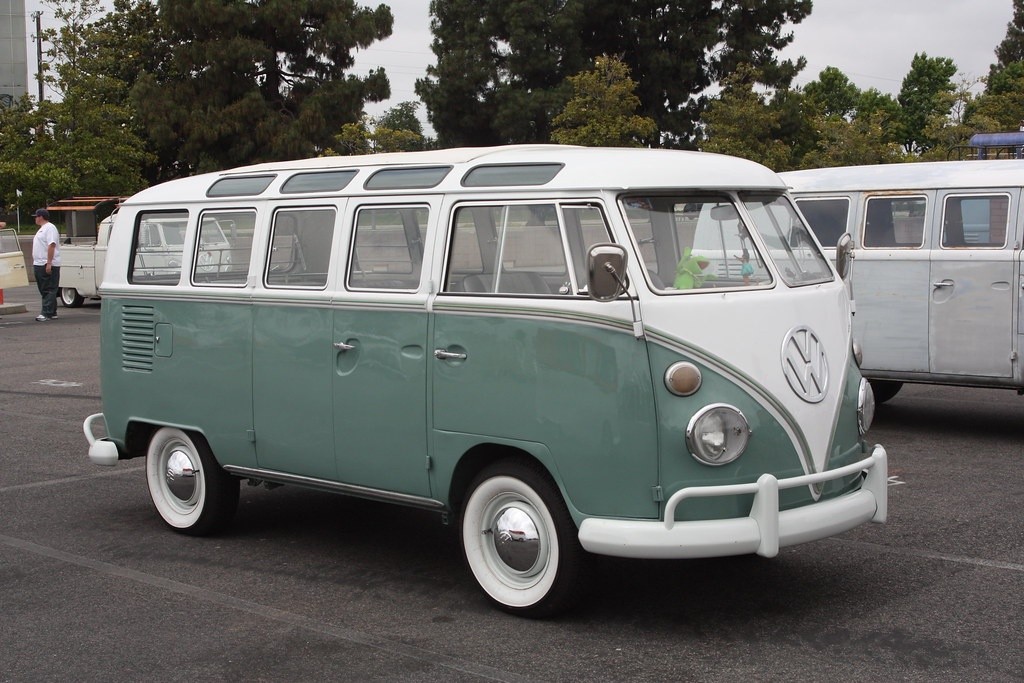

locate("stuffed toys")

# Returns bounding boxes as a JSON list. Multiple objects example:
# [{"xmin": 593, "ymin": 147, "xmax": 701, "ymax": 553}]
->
[{"xmin": 674, "ymin": 246, "xmax": 719, "ymax": 289}]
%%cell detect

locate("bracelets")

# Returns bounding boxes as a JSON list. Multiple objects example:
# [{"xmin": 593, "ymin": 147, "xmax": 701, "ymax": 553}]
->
[{"xmin": 46, "ymin": 262, "xmax": 52, "ymax": 264}]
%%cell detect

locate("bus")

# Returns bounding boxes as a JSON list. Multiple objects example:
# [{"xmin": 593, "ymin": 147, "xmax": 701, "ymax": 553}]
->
[
  {"xmin": 80, "ymin": 141, "xmax": 889, "ymax": 620},
  {"xmin": 691, "ymin": 158, "xmax": 1024, "ymax": 404}
]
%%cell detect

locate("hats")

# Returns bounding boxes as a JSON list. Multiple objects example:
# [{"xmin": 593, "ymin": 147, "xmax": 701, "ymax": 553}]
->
[{"xmin": 31, "ymin": 208, "xmax": 50, "ymax": 220}]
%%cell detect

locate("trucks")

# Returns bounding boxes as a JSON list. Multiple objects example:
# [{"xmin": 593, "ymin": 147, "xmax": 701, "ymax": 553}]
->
[{"xmin": 46, "ymin": 197, "xmax": 237, "ymax": 309}]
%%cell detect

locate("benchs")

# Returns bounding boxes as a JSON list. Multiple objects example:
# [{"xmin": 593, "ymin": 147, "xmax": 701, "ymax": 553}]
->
[{"xmin": 462, "ymin": 271, "xmax": 554, "ymax": 295}]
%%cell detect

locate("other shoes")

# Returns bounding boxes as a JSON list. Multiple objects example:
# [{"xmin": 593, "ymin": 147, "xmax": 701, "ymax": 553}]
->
[
  {"xmin": 51, "ymin": 315, "xmax": 58, "ymax": 319},
  {"xmin": 36, "ymin": 314, "xmax": 51, "ymax": 321}
]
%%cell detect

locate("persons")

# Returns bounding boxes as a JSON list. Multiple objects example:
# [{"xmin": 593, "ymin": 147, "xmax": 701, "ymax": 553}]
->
[
  {"xmin": 734, "ymin": 249, "xmax": 753, "ymax": 285},
  {"xmin": 30, "ymin": 210, "xmax": 61, "ymax": 322}
]
